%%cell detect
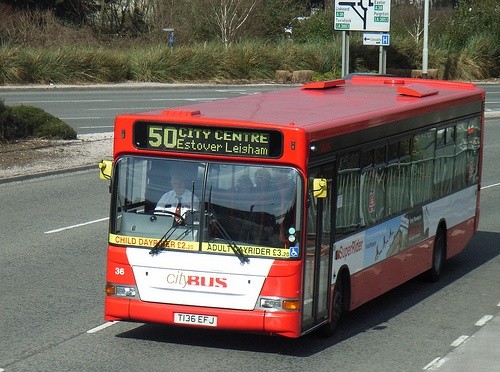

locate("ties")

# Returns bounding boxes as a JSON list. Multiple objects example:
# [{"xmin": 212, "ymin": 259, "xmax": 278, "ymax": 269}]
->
[{"xmin": 174, "ymin": 196, "xmax": 183, "ymax": 225}]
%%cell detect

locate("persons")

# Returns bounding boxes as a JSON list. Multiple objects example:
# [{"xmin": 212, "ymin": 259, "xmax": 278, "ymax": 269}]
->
[
  {"xmin": 236, "ymin": 169, "xmax": 272, "ymax": 194},
  {"xmin": 152, "ymin": 174, "xmax": 200, "ymax": 220}
]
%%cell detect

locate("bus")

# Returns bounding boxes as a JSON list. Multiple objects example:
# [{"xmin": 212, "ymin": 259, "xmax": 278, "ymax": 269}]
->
[{"xmin": 98, "ymin": 75, "xmax": 485, "ymax": 338}]
